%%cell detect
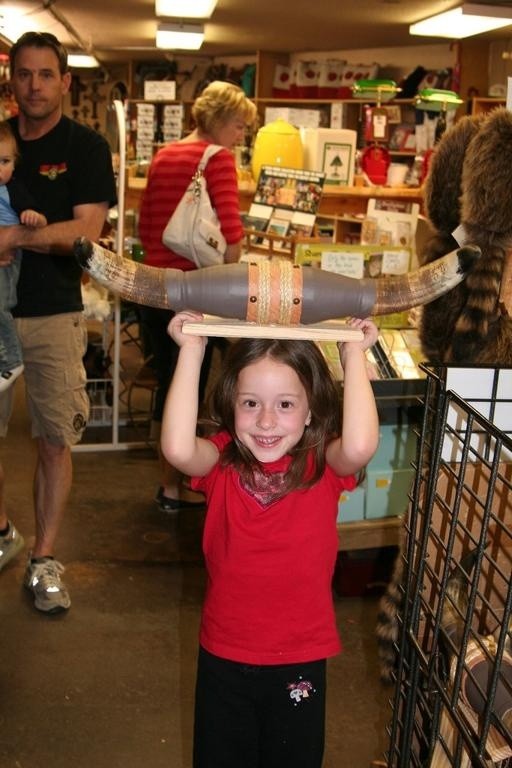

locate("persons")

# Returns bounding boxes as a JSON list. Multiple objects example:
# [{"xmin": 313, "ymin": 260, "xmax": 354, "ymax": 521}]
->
[
  {"xmin": 0, "ymin": 30, "xmax": 118, "ymax": 614},
  {"xmin": 153, "ymin": 306, "xmax": 387, "ymax": 767},
  {"xmin": 0, "ymin": 120, "xmax": 46, "ymax": 398},
  {"xmin": 131, "ymin": 80, "xmax": 260, "ymax": 516}
]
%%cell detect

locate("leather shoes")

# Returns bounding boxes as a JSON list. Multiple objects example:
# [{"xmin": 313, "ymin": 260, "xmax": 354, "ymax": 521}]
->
[{"xmin": 154, "ymin": 482, "xmax": 209, "ymax": 514}]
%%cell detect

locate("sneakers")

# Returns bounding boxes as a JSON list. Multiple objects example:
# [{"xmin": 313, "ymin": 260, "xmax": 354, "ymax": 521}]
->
[
  {"xmin": 22, "ymin": 550, "xmax": 72, "ymax": 613},
  {"xmin": 0, "ymin": 519, "xmax": 26, "ymax": 573},
  {"xmin": 0, "ymin": 362, "xmax": 26, "ymax": 392}
]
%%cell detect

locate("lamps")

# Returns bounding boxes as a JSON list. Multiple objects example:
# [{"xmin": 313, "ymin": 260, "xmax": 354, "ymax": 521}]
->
[
  {"xmin": 154, "ymin": 0, "xmax": 218, "ymax": 51},
  {"xmin": 409, "ymin": 2, "xmax": 512, "ymax": 40},
  {"xmin": 66, "ymin": 49, "xmax": 99, "ymax": 68}
]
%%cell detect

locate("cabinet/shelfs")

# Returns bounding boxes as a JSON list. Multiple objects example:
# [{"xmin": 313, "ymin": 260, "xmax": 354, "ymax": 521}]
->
[{"xmin": 111, "ymin": 41, "xmax": 512, "ymax": 554}]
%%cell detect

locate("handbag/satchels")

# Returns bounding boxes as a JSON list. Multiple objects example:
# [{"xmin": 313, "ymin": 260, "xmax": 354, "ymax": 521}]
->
[{"xmin": 159, "ymin": 142, "xmax": 238, "ymax": 270}]
